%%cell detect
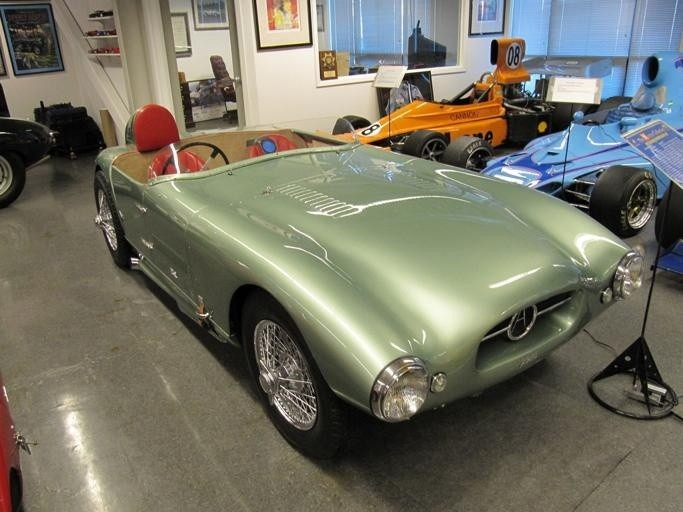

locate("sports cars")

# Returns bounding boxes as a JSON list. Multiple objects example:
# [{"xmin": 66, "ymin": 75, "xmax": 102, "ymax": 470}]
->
[{"xmin": 93, "ymin": 104, "xmax": 646, "ymax": 460}]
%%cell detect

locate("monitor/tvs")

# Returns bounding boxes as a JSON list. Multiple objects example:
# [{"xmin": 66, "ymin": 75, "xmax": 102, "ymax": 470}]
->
[{"xmin": 408, "ymin": 34, "xmax": 447, "ymax": 68}]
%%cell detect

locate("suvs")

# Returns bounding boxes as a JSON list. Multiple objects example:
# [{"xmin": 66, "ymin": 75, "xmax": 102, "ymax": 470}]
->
[
  {"xmin": 204, "ymin": 4, "xmax": 220, "ymax": 18},
  {"xmin": 11, "ymin": 12, "xmax": 48, "ymax": 69}
]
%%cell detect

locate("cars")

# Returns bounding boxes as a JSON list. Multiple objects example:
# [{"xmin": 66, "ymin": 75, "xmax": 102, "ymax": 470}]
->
[
  {"xmin": 0, "ymin": 374, "xmax": 37, "ymax": 512},
  {"xmin": 0, "ymin": 84, "xmax": 59, "ymax": 207}
]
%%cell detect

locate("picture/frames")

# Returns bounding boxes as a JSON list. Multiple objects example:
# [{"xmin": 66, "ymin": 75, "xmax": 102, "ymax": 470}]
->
[
  {"xmin": 191, "ymin": 0, "xmax": 231, "ymax": 30},
  {"xmin": 170, "ymin": 12, "xmax": 193, "ymax": 58},
  {"xmin": 467, "ymin": 0, "xmax": 508, "ymax": 38},
  {"xmin": 252, "ymin": 0, "xmax": 313, "ymax": 53},
  {"xmin": 0, "ymin": 3, "xmax": 65, "ymax": 77}
]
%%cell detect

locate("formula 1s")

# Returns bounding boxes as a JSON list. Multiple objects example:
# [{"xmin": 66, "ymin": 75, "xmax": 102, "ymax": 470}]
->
[{"xmin": 332, "ymin": 38, "xmax": 682, "ymax": 249}]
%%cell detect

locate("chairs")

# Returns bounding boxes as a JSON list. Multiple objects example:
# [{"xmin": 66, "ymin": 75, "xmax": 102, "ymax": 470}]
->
[
  {"xmin": 115, "ymin": 104, "xmax": 209, "ymax": 181},
  {"xmin": 603, "ymin": 92, "xmax": 663, "ymax": 126},
  {"xmin": 210, "ymin": 55, "xmax": 237, "ymax": 118}
]
